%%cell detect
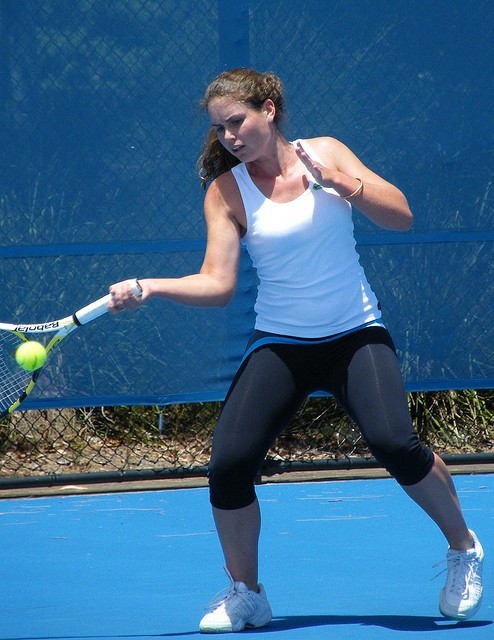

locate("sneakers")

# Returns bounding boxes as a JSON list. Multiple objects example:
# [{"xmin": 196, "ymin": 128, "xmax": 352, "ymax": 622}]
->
[
  {"xmin": 430, "ymin": 529, "xmax": 484, "ymax": 619},
  {"xmin": 199, "ymin": 566, "xmax": 272, "ymax": 633}
]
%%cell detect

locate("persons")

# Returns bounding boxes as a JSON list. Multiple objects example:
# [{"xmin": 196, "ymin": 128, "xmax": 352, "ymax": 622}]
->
[{"xmin": 108, "ymin": 68, "xmax": 484, "ymax": 634}]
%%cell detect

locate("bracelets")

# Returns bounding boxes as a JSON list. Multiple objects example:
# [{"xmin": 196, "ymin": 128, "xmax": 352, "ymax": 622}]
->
[{"xmin": 340, "ymin": 177, "xmax": 364, "ymax": 201}]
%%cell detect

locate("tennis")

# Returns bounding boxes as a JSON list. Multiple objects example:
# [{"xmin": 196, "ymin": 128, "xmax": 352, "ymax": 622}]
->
[{"xmin": 15, "ymin": 340, "xmax": 47, "ymax": 372}]
